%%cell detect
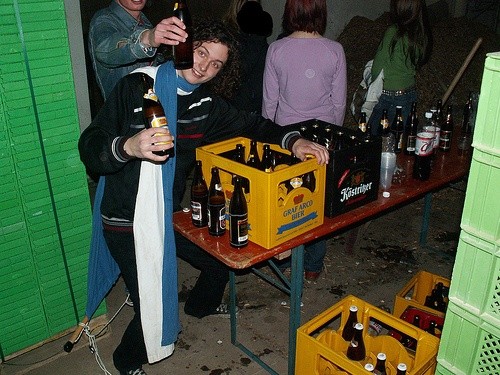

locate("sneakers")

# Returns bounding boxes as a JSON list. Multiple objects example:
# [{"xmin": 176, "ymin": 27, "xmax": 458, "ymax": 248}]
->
[
  {"xmin": 206, "ymin": 303, "xmax": 240, "ymax": 319},
  {"xmin": 119, "ymin": 364, "xmax": 147, "ymax": 375}
]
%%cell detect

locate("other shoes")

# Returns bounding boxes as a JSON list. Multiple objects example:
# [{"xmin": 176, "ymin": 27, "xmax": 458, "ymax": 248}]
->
[{"xmin": 305, "ymin": 269, "xmax": 322, "ymax": 281}]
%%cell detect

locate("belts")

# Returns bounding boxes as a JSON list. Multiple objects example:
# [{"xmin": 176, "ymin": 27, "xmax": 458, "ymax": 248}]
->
[{"xmin": 383, "ymin": 86, "xmax": 419, "ymax": 96}]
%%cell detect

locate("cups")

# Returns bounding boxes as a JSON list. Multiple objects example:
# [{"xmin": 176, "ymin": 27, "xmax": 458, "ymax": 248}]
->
[{"xmin": 378, "ymin": 151, "xmax": 396, "ymax": 188}]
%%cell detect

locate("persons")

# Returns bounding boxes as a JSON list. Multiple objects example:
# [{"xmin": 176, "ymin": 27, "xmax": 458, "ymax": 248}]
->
[
  {"xmin": 223, "ymin": 0, "xmax": 273, "ymax": 115},
  {"xmin": 88, "ymin": 0, "xmax": 189, "ymax": 102},
  {"xmin": 78, "ymin": 23, "xmax": 329, "ymax": 375},
  {"xmin": 367, "ymin": 0, "xmax": 427, "ymax": 133},
  {"xmin": 262, "ymin": 0, "xmax": 347, "ymax": 280}
]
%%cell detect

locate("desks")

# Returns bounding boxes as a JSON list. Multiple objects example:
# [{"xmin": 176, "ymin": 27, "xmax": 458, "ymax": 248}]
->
[{"xmin": 173, "ymin": 120, "xmax": 474, "ymax": 375}]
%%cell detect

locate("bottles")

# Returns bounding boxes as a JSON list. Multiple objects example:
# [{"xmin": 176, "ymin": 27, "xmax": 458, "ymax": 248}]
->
[
  {"xmin": 461, "ymin": 97, "xmax": 475, "ymax": 134},
  {"xmin": 230, "ymin": 112, "xmax": 371, "ymax": 192},
  {"xmin": 375, "ymin": 352, "xmax": 387, "ymax": 375},
  {"xmin": 436, "ymin": 99, "xmax": 443, "ymax": 125},
  {"xmin": 396, "ymin": 363, "xmax": 407, "ymax": 375},
  {"xmin": 425, "ymin": 321, "xmax": 436, "ymax": 336},
  {"xmin": 412, "ymin": 112, "xmax": 435, "ymax": 180},
  {"xmin": 429, "ymin": 106, "xmax": 440, "ymax": 167},
  {"xmin": 376, "ymin": 108, "xmax": 390, "ymax": 152},
  {"xmin": 391, "ymin": 106, "xmax": 406, "ymax": 152},
  {"xmin": 403, "ymin": 315, "xmax": 421, "ymax": 348},
  {"xmin": 190, "ymin": 160, "xmax": 208, "ymax": 227},
  {"xmin": 140, "ymin": 74, "xmax": 175, "ymax": 157},
  {"xmin": 363, "ymin": 363, "xmax": 375, "ymax": 373},
  {"xmin": 207, "ymin": 167, "xmax": 227, "ymax": 237},
  {"xmin": 423, "ymin": 279, "xmax": 450, "ymax": 311},
  {"xmin": 229, "ymin": 176, "xmax": 250, "ymax": 247},
  {"xmin": 440, "ymin": 106, "xmax": 454, "ymax": 152},
  {"xmin": 173, "ymin": 0, "xmax": 194, "ymax": 70},
  {"xmin": 347, "ymin": 323, "xmax": 366, "ymax": 360},
  {"xmin": 341, "ymin": 306, "xmax": 358, "ymax": 341},
  {"xmin": 406, "ymin": 102, "xmax": 420, "ymax": 155}
]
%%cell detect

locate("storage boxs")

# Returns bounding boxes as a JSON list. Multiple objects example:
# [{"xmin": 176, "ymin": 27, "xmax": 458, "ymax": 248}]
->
[{"xmin": 196, "ymin": 51, "xmax": 500, "ymax": 375}]
